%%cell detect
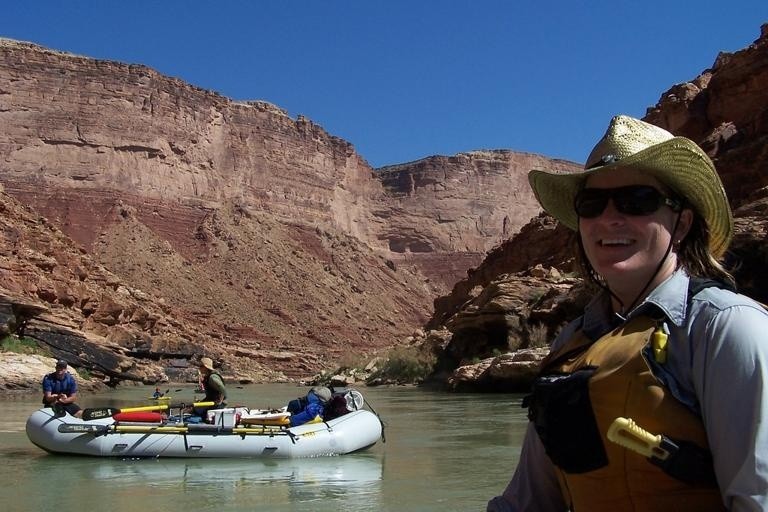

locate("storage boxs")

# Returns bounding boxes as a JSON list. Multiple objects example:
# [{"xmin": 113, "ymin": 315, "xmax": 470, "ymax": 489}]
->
[{"xmin": 214, "ymin": 407, "xmax": 247, "ymax": 428}]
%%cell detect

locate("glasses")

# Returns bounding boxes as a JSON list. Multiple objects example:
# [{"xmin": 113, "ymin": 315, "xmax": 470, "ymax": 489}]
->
[{"xmin": 573, "ymin": 185, "xmax": 682, "ymax": 219}]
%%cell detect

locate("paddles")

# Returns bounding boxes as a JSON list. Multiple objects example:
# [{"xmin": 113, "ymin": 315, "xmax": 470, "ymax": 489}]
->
[
  {"xmin": 58, "ymin": 424, "xmax": 291, "ymax": 433},
  {"xmin": 83, "ymin": 400, "xmax": 227, "ymax": 421},
  {"xmin": 161, "ymin": 389, "xmax": 170, "ymax": 397}
]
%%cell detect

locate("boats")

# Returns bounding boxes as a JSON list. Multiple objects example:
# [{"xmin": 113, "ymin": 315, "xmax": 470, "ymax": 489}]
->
[
  {"xmin": 330, "ymin": 379, "xmax": 353, "ymax": 388},
  {"xmin": 194, "ymin": 384, "xmax": 205, "ymax": 393},
  {"xmin": 92, "ymin": 454, "xmax": 386, "ymax": 483},
  {"xmin": 24, "ymin": 404, "xmax": 385, "ymax": 460},
  {"xmin": 148, "ymin": 397, "xmax": 171, "ymax": 401}
]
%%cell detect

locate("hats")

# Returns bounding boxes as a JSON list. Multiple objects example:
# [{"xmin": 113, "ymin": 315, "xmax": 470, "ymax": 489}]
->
[
  {"xmin": 56, "ymin": 359, "xmax": 67, "ymax": 368},
  {"xmin": 528, "ymin": 115, "xmax": 734, "ymax": 261},
  {"xmin": 200, "ymin": 357, "xmax": 215, "ymax": 370}
]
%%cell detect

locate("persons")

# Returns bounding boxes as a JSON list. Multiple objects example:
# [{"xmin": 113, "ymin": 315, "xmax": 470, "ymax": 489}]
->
[
  {"xmin": 39, "ymin": 358, "xmax": 83, "ymax": 421},
  {"xmin": 198, "ymin": 357, "xmax": 228, "ymax": 422},
  {"xmin": 486, "ymin": 114, "xmax": 767, "ymax": 512},
  {"xmin": 152, "ymin": 387, "xmax": 164, "ymax": 399}
]
